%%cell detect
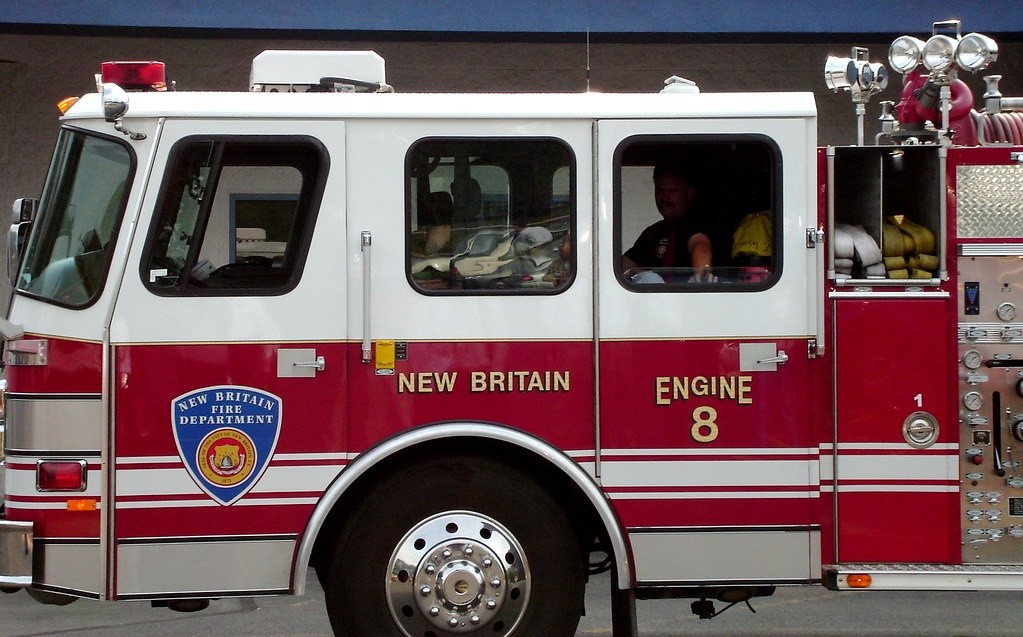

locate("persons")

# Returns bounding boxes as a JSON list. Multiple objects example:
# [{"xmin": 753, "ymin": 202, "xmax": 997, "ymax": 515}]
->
[
  {"xmin": 507, "ymin": 196, "xmax": 554, "ymax": 286},
  {"xmin": 451, "ymin": 179, "xmax": 481, "ymax": 235},
  {"xmin": 621, "ymin": 161, "xmax": 718, "ymax": 284}
]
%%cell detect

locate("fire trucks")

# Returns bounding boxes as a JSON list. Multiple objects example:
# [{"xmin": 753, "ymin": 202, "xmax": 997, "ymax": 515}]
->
[{"xmin": 0, "ymin": 15, "xmax": 1023, "ymax": 636}]
